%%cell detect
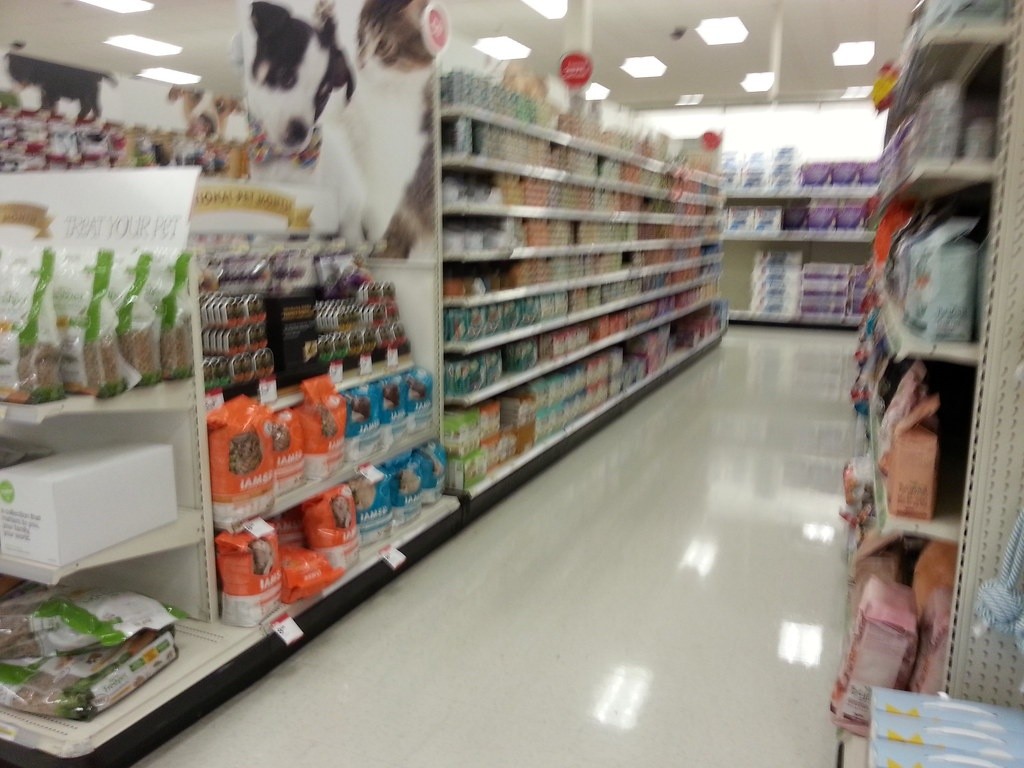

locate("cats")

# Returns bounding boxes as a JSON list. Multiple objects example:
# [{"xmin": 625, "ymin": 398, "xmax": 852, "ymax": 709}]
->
[{"xmin": 337, "ymin": 0, "xmax": 439, "ymax": 266}]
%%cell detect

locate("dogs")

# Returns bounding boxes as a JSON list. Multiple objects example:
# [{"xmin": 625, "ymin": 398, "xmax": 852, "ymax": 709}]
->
[
  {"xmin": 167, "ymin": 84, "xmax": 240, "ymax": 144},
  {"xmin": 5, "ymin": 52, "xmax": 116, "ymax": 126},
  {"xmin": 244, "ymin": 2, "xmax": 370, "ymax": 256}
]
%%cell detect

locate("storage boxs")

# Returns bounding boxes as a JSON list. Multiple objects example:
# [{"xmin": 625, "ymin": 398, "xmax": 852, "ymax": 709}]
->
[
  {"xmin": 751, "ymin": 280, "xmax": 800, "ymax": 296},
  {"xmin": 754, "ymin": 205, "xmax": 781, "ymax": 230},
  {"xmin": 0, "ymin": 439, "xmax": 180, "ymax": 568},
  {"xmin": 784, "ymin": 207, "xmax": 808, "ymax": 231},
  {"xmin": 836, "ymin": 206, "xmax": 864, "ymax": 230},
  {"xmin": 831, "ymin": 162, "xmax": 857, "ymax": 189},
  {"xmin": 743, "ymin": 150, "xmax": 771, "ymax": 175},
  {"xmin": 751, "ymin": 295, "xmax": 800, "ymax": 314},
  {"xmin": 754, "ymin": 249, "xmax": 803, "ymax": 265},
  {"xmin": 729, "ymin": 206, "xmax": 753, "ymax": 231},
  {"xmin": 800, "ymin": 295, "xmax": 849, "ymax": 318},
  {"xmin": 721, "ymin": 151, "xmax": 743, "ymax": 175},
  {"xmin": 803, "ymin": 260, "xmax": 852, "ymax": 278},
  {"xmin": 802, "ymin": 162, "xmax": 831, "ymax": 188},
  {"xmin": 742, "ymin": 173, "xmax": 770, "ymax": 190},
  {"xmin": 808, "ymin": 207, "xmax": 837, "ymax": 230},
  {"xmin": 722, "ymin": 173, "xmax": 741, "ymax": 190},
  {"xmin": 751, "ymin": 264, "xmax": 802, "ymax": 280},
  {"xmin": 802, "ymin": 279, "xmax": 849, "ymax": 297},
  {"xmin": 858, "ymin": 162, "xmax": 879, "ymax": 188}
]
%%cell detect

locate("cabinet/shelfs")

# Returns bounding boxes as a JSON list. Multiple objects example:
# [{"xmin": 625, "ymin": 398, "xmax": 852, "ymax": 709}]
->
[{"xmin": 0, "ymin": 0, "xmax": 1024, "ymax": 768}]
